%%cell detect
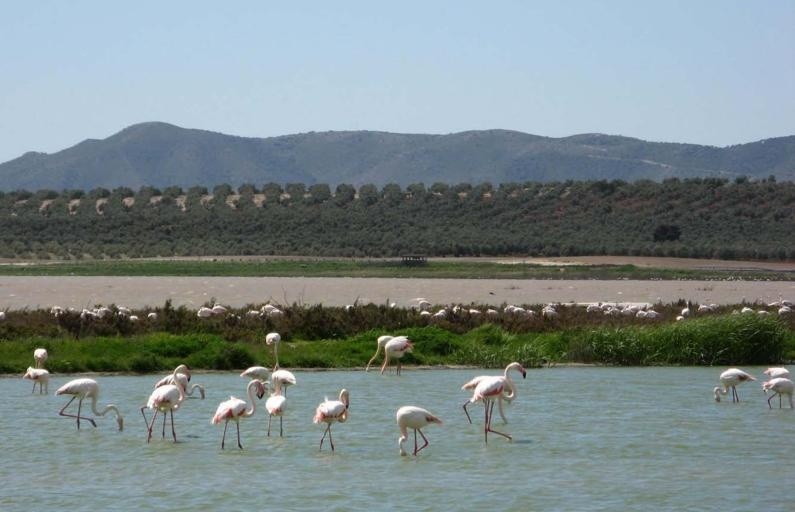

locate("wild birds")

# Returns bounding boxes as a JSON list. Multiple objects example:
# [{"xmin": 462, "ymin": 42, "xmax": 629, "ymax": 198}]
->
[
  {"xmin": 140, "ymin": 362, "xmax": 296, "ymax": 452},
  {"xmin": 312, "ymin": 387, "xmax": 351, "ymax": 452},
  {"xmin": 461, "ymin": 360, "xmax": 527, "ymax": 445},
  {"xmin": 21, "ymin": 347, "xmax": 51, "ymax": 395},
  {"xmin": 396, "ymin": 405, "xmax": 443, "ymax": 457},
  {"xmin": 54, "ymin": 377, "xmax": 124, "ymax": 433},
  {"xmin": 713, "ymin": 366, "xmax": 794, "ymax": 410},
  {"xmin": 50, "ymin": 300, "xmax": 285, "ymax": 322},
  {"xmin": 265, "ymin": 331, "xmax": 281, "ymax": 371},
  {"xmin": 412, "ymin": 293, "xmax": 795, "ymax": 322},
  {"xmin": 366, "ymin": 335, "xmax": 413, "ymax": 377}
]
